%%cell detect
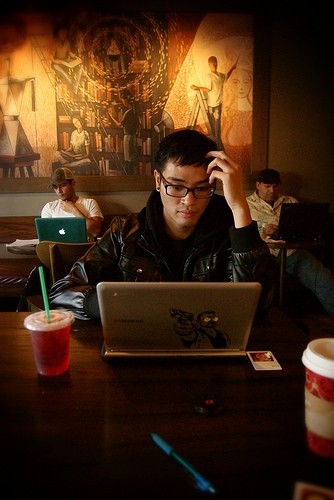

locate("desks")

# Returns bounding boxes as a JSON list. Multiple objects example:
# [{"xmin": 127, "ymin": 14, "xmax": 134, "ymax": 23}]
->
[
  {"xmin": 0, "ymin": 238, "xmax": 324, "ymax": 308},
  {"xmin": 0, "ymin": 312, "xmax": 334, "ymax": 500}
]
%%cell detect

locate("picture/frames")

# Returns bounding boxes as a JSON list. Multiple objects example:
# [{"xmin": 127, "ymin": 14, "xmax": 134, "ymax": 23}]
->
[{"xmin": 0, "ymin": 0, "xmax": 274, "ymax": 195}]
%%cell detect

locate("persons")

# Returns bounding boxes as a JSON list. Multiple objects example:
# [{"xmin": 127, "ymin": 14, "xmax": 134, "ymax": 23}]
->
[
  {"xmin": 48, "ymin": 129, "xmax": 277, "ymax": 327},
  {"xmin": 17, "ymin": 168, "xmax": 104, "ymax": 312},
  {"xmin": 244, "ymin": 169, "xmax": 334, "ymax": 318}
]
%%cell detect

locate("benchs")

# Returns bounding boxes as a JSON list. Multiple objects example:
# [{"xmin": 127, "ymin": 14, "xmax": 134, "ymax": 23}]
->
[{"xmin": 0, "ymin": 213, "xmax": 140, "ymax": 299}]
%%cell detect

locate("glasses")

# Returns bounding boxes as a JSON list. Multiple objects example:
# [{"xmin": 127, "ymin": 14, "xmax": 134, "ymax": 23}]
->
[{"xmin": 158, "ymin": 171, "xmax": 216, "ymax": 199}]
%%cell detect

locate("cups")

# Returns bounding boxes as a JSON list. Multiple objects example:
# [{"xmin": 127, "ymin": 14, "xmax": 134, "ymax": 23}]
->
[
  {"xmin": 257, "ymin": 220, "xmax": 268, "ymax": 240},
  {"xmin": 23, "ymin": 310, "xmax": 75, "ymax": 376},
  {"xmin": 301, "ymin": 337, "xmax": 334, "ymax": 458}
]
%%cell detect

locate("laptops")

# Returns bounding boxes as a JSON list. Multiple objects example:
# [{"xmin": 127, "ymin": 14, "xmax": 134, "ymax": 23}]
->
[
  {"xmin": 271, "ymin": 202, "xmax": 331, "ymax": 243},
  {"xmin": 35, "ymin": 217, "xmax": 88, "ymax": 243},
  {"xmin": 96, "ymin": 282, "xmax": 262, "ymax": 359}
]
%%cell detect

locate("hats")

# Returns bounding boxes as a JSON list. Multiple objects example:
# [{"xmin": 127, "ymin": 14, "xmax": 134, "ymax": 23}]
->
[
  {"xmin": 258, "ymin": 168, "xmax": 281, "ymax": 184},
  {"xmin": 47, "ymin": 167, "xmax": 75, "ymax": 188}
]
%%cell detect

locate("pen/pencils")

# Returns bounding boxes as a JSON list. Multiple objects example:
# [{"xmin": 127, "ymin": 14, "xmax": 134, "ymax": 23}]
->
[{"xmin": 150, "ymin": 431, "xmax": 215, "ymax": 493}]
